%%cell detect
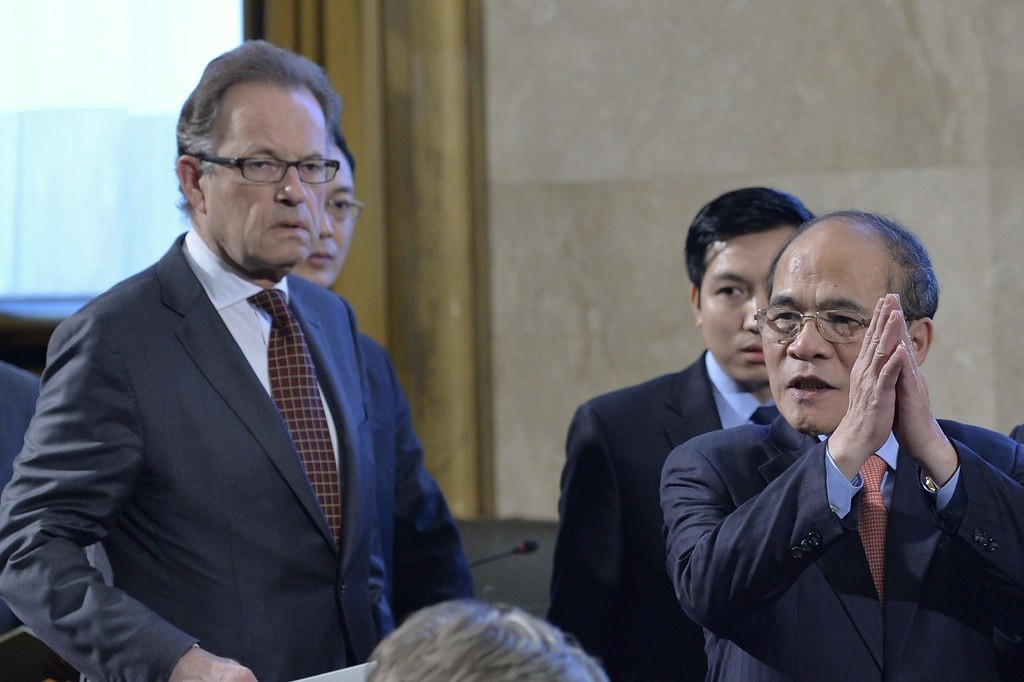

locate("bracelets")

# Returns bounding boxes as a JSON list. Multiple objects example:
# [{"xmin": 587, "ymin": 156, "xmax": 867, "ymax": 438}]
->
[{"xmin": 926, "ymin": 469, "xmax": 942, "ymax": 491}]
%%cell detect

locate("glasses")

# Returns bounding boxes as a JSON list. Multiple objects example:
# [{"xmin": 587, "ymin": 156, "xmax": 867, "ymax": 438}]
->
[
  {"xmin": 750, "ymin": 305, "xmax": 868, "ymax": 346},
  {"xmin": 187, "ymin": 154, "xmax": 341, "ymax": 184},
  {"xmin": 326, "ymin": 197, "xmax": 363, "ymax": 222}
]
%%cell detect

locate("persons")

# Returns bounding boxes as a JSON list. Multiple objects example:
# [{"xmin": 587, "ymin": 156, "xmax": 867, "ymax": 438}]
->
[
  {"xmin": 0, "ymin": 42, "xmax": 391, "ymax": 682},
  {"xmin": 288, "ymin": 129, "xmax": 476, "ymax": 623},
  {"xmin": 367, "ymin": 597, "xmax": 607, "ymax": 682},
  {"xmin": 659, "ymin": 209, "xmax": 1024, "ymax": 682},
  {"xmin": 547, "ymin": 185, "xmax": 815, "ymax": 682}
]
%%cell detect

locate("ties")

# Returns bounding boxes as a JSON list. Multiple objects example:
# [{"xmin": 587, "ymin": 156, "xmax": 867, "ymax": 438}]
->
[
  {"xmin": 246, "ymin": 287, "xmax": 340, "ymax": 543},
  {"xmin": 856, "ymin": 452, "xmax": 887, "ymax": 601}
]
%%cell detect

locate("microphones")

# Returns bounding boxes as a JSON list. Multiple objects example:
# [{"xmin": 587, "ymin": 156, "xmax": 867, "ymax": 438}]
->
[{"xmin": 469, "ymin": 540, "xmax": 539, "ymax": 569}]
